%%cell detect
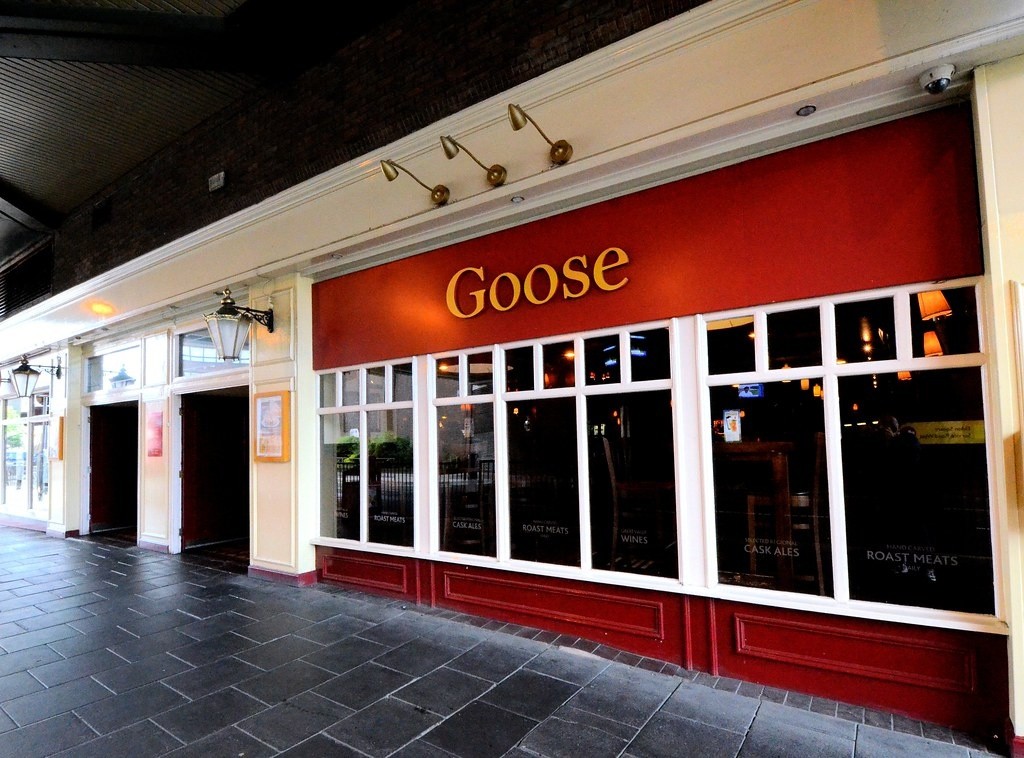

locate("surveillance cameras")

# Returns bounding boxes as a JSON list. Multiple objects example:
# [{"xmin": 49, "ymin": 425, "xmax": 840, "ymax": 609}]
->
[{"xmin": 917, "ymin": 63, "xmax": 956, "ymax": 94}]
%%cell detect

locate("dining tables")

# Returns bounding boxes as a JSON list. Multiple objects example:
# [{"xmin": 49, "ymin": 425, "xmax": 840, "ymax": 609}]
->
[{"xmin": 713, "ymin": 439, "xmax": 795, "ymax": 580}]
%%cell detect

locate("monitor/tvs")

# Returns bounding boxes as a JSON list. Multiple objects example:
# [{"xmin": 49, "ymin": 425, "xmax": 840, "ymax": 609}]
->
[{"xmin": 737, "ymin": 383, "xmax": 764, "ymax": 398}]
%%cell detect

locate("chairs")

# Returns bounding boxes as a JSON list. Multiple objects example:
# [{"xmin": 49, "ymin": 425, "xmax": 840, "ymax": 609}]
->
[
  {"xmin": 602, "ymin": 436, "xmax": 677, "ymax": 576},
  {"xmin": 443, "ymin": 459, "xmax": 495, "ymax": 556},
  {"xmin": 747, "ymin": 431, "xmax": 826, "ymax": 597}
]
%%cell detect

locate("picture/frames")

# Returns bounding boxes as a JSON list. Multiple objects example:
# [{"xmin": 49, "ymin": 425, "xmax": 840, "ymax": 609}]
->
[
  {"xmin": 48, "ymin": 416, "xmax": 63, "ymax": 461},
  {"xmin": 253, "ymin": 391, "xmax": 290, "ymax": 462},
  {"xmin": 142, "ymin": 396, "xmax": 170, "ymax": 460}
]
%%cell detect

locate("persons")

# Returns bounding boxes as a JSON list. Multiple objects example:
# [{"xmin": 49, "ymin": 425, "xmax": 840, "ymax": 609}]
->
[{"xmin": 856, "ymin": 416, "xmax": 921, "ymax": 445}]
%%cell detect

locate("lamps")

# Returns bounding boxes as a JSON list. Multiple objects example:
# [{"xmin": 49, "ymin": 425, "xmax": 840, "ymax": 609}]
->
[
  {"xmin": 380, "ymin": 159, "xmax": 450, "ymax": 205},
  {"xmin": 439, "ymin": 134, "xmax": 507, "ymax": 187},
  {"xmin": 507, "ymin": 103, "xmax": 574, "ymax": 165},
  {"xmin": 7, "ymin": 353, "xmax": 40, "ymax": 398},
  {"xmin": 898, "ymin": 371, "xmax": 911, "ymax": 380},
  {"xmin": 202, "ymin": 289, "xmax": 253, "ymax": 361},
  {"xmin": 782, "ymin": 365, "xmax": 824, "ymax": 399},
  {"xmin": 923, "ymin": 330, "xmax": 943, "ymax": 357},
  {"xmin": 917, "ymin": 291, "xmax": 952, "ymax": 320}
]
